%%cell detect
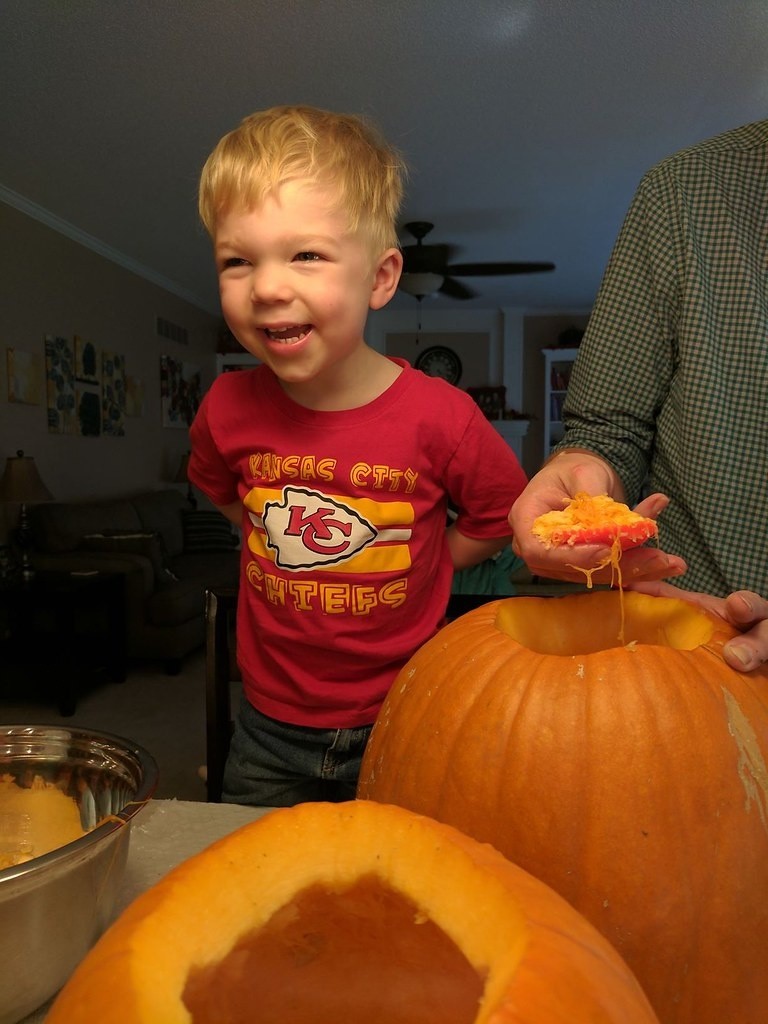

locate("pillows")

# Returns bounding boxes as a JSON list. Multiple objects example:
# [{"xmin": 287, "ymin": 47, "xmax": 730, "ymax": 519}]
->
[
  {"xmin": 182, "ymin": 507, "xmax": 234, "ymax": 556},
  {"xmin": 102, "ymin": 529, "xmax": 154, "ymax": 557}
]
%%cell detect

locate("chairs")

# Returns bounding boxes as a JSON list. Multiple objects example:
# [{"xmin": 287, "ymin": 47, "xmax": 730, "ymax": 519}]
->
[{"xmin": 199, "ymin": 586, "xmax": 552, "ymax": 812}]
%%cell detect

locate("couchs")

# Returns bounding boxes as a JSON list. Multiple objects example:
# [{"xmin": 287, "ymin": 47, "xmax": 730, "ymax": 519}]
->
[{"xmin": 30, "ymin": 490, "xmax": 241, "ymax": 672}]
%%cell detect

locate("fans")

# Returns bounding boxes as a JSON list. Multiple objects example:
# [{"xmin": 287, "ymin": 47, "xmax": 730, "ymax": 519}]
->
[{"xmin": 398, "ymin": 222, "xmax": 556, "ymax": 345}]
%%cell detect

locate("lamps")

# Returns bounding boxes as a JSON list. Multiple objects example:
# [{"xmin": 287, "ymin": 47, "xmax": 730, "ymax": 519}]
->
[
  {"xmin": 168, "ymin": 450, "xmax": 198, "ymax": 510},
  {"xmin": 398, "ymin": 271, "xmax": 444, "ymax": 345},
  {"xmin": 0, "ymin": 450, "xmax": 56, "ymax": 582}
]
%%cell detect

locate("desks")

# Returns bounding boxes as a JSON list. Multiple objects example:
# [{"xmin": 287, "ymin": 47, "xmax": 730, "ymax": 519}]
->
[{"xmin": 0, "ymin": 798, "xmax": 292, "ymax": 1024}]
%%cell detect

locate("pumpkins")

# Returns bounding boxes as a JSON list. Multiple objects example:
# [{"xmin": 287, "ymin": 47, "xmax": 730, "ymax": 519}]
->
[
  {"xmin": 355, "ymin": 591, "xmax": 768, "ymax": 1024},
  {"xmin": 44, "ymin": 798, "xmax": 663, "ymax": 1024}
]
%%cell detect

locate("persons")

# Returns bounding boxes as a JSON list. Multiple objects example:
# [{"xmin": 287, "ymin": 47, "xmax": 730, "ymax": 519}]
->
[
  {"xmin": 185, "ymin": 105, "xmax": 530, "ymax": 810},
  {"xmin": 507, "ymin": 117, "xmax": 768, "ymax": 672}
]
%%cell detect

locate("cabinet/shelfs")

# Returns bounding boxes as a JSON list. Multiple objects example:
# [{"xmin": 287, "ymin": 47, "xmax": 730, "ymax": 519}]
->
[
  {"xmin": 541, "ymin": 349, "xmax": 578, "ymax": 460},
  {"xmin": 467, "ymin": 386, "xmax": 505, "ymax": 419}
]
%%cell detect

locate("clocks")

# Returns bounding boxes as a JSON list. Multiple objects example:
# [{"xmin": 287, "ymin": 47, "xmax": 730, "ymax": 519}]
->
[{"xmin": 413, "ymin": 345, "xmax": 462, "ymax": 387}]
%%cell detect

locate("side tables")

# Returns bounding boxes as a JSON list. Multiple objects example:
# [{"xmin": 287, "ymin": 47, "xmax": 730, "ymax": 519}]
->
[{"xmin": 0, "ymin": 572, "xmax": 131, "ymax": 717}]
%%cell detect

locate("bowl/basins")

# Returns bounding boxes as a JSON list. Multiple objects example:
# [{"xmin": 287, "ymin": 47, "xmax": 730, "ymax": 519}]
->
[{"xmin": 0, "ymin": 725, "xmax": 159, "ymax": 1024}]
